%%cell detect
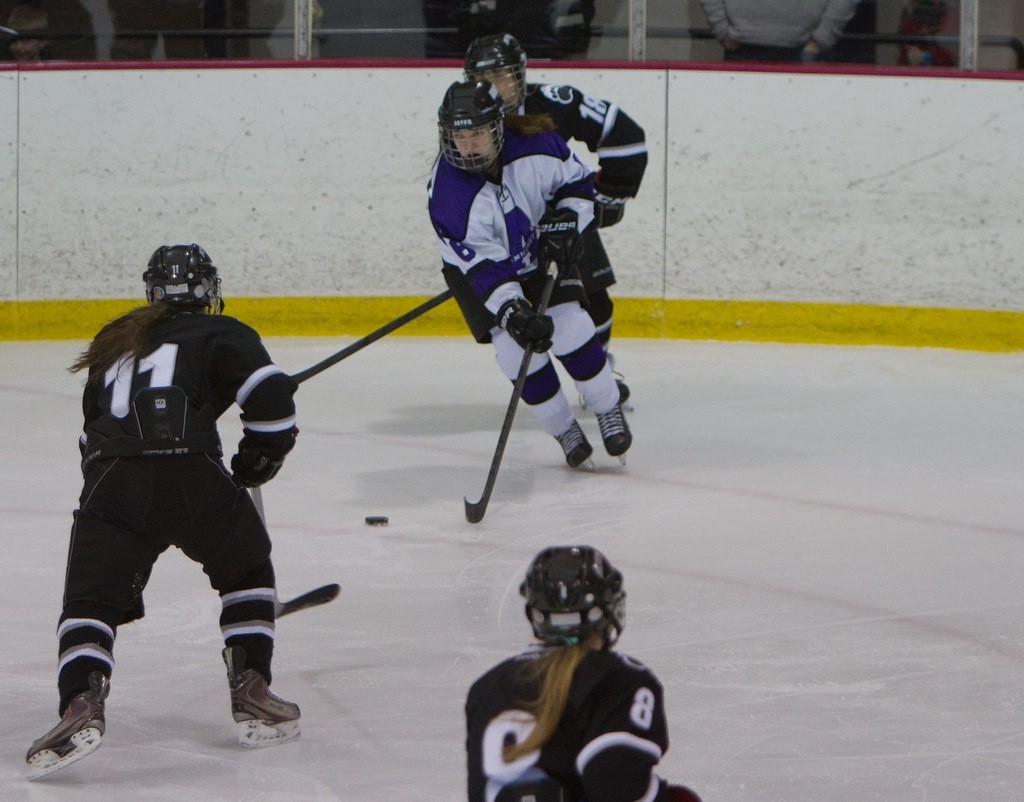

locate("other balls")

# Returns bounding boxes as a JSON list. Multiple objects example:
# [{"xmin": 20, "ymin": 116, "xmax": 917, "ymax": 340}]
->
[{"xmin": 366, "ymin": 516, "xmax": 389, "ymax": 527}]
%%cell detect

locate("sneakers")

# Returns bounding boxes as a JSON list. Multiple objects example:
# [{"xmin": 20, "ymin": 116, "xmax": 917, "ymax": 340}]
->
[
  {"xmin": 26, "ymin": 671, "xmax": 110, "ymax": 781},
  {"xmin": 595, "ymin": 403, "xmax": 633, "ymax": 466},
  {"xmin": 222, "ymin": 647, "xmax": 301, "ymax": 747},
  {"xmin": 554, "ymin": 419, "xmax": 595, "ymax": 473},
  {"xmin": 579, "ymin": 353, "xmax": 630, "ymax": 410}
]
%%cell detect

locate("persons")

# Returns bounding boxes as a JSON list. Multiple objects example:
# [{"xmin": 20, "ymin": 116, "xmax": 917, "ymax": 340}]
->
[
  {"xmin": 426, "ymin": 78, "xmax": 636, "ymax": 472},
  {"xmin": 701, "ymin": 0, "xmax": 860, "ymax": 62},
  {"xmin": 3, "ymin": 2, "xmax": 223, "ymax": 67},
  {"xmin": 465, "ymin": 544, "xmax": 701, "ymax": 801},
  {"xmin": 23, "ymin": 242, "xmax": 304, "ymax": 777},
  {"xmin": 420, "ymin": 0, "xmax": 595, "ymax": 61},
  {"xmin": 458, "ymin": 33, "xmax": 649, "ymax": 410}
]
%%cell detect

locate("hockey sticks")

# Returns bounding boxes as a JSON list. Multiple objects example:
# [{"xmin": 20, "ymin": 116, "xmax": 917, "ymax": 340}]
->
[
  {"xmin": 252, "ymin": 486, "xmax": 340, "ymax": 620},
  {"xmin": 288, "ymin": 290, "xmax": 454, "ymax": 383},
  {"xmin": 462, "ymin": 252, "xmax": 559, "ymax": 523}
]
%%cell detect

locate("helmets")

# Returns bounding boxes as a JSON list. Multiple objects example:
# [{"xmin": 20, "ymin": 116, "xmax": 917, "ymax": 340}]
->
[
  {"xmin": 143, "ymin": 243, "xmax": 224, "ymax": 315},
  {"xmin": 464, "ymin": 34, "xmax": 527, "ymax": 114},
  {"xmin": 520, "ymin": 545, "xmax": 626, "ymax": 640},
  {"xmin": 437, "ymin": 80, "xmax": 505, "ymax": 169}
]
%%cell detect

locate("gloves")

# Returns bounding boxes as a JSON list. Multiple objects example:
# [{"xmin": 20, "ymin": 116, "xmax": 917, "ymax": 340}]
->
[
  {"xmin": 594, "ymin": 177, "xmax": 632, "ymax": 227},
  {"xmin": 231, "ymin": 436, "xmax": 286, "ymax": 488},
  {"xmin": 496, "ymin": 299, "xmax": 553, "ymax": 353},
  {"xmin": 539, "ymin": 208, "xmax": 585, "ymax": 270}
]
